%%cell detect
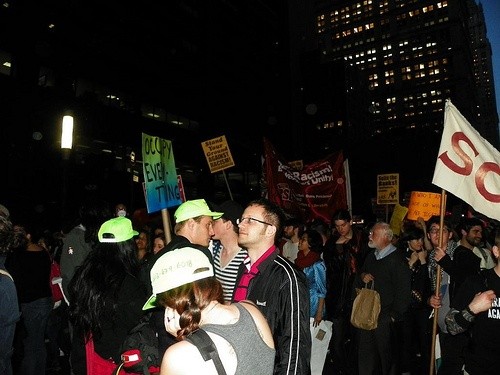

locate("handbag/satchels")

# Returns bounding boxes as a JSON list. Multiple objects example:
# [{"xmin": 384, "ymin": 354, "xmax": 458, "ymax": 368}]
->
[
  {"xmin": 116, "ymin": 323, "xmax": 159, "ymax": 374},
  {"xmin": 350, "ymin": 280, "xmax": 382, "ymax": 330}
]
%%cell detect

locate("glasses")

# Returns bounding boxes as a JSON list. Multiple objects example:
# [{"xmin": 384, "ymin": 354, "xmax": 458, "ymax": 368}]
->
[
  {"xmin": 428, "ymin": 226, "xmax": 450, "ymax": 234},
  {"xmin": 235, "ymin": 217, "xmax": 272, "ymax": 226}
]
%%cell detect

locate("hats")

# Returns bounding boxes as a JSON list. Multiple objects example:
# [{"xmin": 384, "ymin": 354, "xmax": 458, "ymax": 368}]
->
[
  {"xmin": 98, "ymin": 216, "xmax": 139, "ymax": 243},
  {"xmin": 142, "ymin": 247, "xmax": 214, "ymax": 312},
  {"xmin": 174, "ymin": 199, "xmax": 225, "ymax": 221}
]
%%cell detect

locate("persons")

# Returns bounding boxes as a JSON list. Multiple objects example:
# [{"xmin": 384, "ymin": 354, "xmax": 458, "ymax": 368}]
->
[{"xmin": 0, "ymin": 197, "xmax": 500, "ymax": 375}]
